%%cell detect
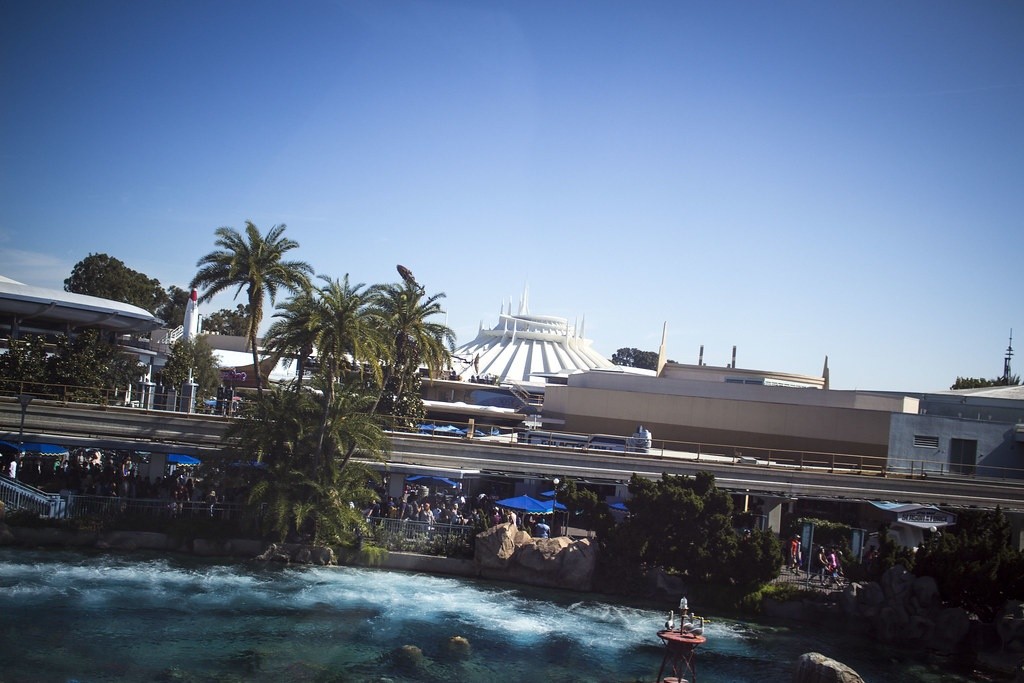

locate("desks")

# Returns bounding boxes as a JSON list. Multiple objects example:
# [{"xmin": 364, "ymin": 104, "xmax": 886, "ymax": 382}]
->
[{"xmin": 656, "ymin": 630, "xmax": 707, "ymax": 683}]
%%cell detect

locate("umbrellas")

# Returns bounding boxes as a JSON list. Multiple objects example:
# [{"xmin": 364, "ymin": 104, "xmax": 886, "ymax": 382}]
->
[
  {"xmin": 229, "ymin": 460, "xmax": 269, "ymax": 470},
  {"xmin": 0, "ymin": 440, "xmax": 69, "ymax": 456},
  {"xmin": 542, "ymin": 499, "xmax": 568, "ymax": 533},
  {"xmin": 404, "ymin": 475, "xmax": 458, "ymax": 494},
  {"xmin": 183, "ymin": 285, "xmax": 198, "ymax": 383},
  {"xmin": 539, "ymin": 491, "xmax": 556, "ymax": 499},
  {"xmin": 166, "ymin": 452, "xmax": 202, "ymax": 471},
  {"xmin": 494, "ymin": 493, "xmax": 554, "ymax": 530},
  {"xmin": 609, "ymin": 502, "xmax": 630, "ymax": 512}
]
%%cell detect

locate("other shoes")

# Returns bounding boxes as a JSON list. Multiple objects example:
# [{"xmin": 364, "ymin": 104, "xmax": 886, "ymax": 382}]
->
[
  {"xmin": 790, "ymin": 570, "xmax": 796, "ymax": 573},
  {"xmin": 796, "ymin": 573, "xmax": 801, "ymax": 576}
]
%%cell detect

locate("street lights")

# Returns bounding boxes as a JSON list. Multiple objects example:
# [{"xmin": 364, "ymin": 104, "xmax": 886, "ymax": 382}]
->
[
  {"xmin": 15, "ymin": 395, "xmax": 37, "ymax": 481},
  {"xmin": 553, "ymin": 478, "xmax": 559, "ymax": 513}
]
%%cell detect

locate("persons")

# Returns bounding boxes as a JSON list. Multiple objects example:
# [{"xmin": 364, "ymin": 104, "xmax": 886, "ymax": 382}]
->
[
  {"xmin": 470, "ymin": 375, "xmax": 479, "ymax": 383},
  {"xmin": 786, "ymin": 533, "xmax": 803, "ymax": 576},
  {"xmin": 571, "ymin": 510, "xmax": 612, "ymax": 539},
  {"xmin": 870, "ymin": 545, "xmax": 881, "ymax": 558},
  {"xmin": 357, "ymin": 483, "xmax": 551, "ymax": 540},
  {"xmin": 0, "ymin": 447, "xmax": 218, "ymax": 520},
  {"xmin": 810, "ymin": 546, "xmax": 844, "ymax": 586},
  {"xmin": 449, "ymin": 371, "xmax": 460, "ymax": 379}
]
